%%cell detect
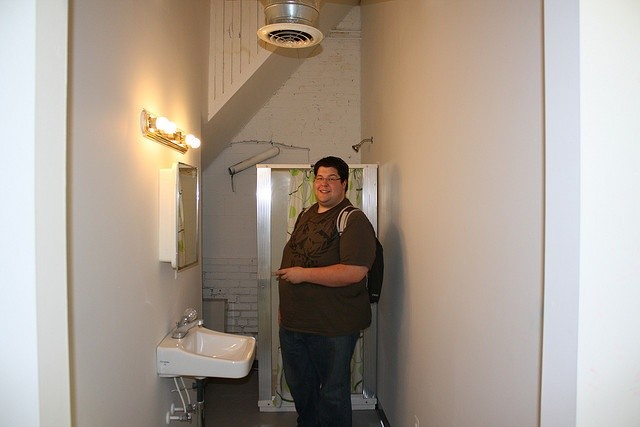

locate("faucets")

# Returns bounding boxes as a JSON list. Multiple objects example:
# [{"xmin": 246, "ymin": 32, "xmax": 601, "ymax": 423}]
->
[{"xmin": 173, "ymin": 312, "xmax": 204, "ymax": 339}]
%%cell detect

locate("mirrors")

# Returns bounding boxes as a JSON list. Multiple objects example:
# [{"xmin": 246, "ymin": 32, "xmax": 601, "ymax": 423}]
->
[{"xmin": 176, "ymin": 163, "xmax": 199, "ymax": 270}]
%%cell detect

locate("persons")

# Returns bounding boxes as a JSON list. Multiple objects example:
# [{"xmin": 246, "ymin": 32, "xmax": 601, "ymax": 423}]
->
[{"xmin": 272, "ymin": 156, "xmax": 378, "ymax": 426}]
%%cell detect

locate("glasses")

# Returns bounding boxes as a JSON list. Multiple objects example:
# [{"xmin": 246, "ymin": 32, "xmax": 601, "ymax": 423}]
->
[{"xmin": 314, "ymin": 176, "xmax": 341, "ymax": 182}]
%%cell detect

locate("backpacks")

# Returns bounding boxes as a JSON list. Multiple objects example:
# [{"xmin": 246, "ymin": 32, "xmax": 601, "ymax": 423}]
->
[{"xmin": 301, "ymin": 205, "xmax": 384, "ymax": 304}]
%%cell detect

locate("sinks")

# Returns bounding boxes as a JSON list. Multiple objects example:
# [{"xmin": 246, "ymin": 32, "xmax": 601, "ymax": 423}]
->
[{"xmin": 157, "ymin": 325, "xmax": 256, "ymax": 379}]
